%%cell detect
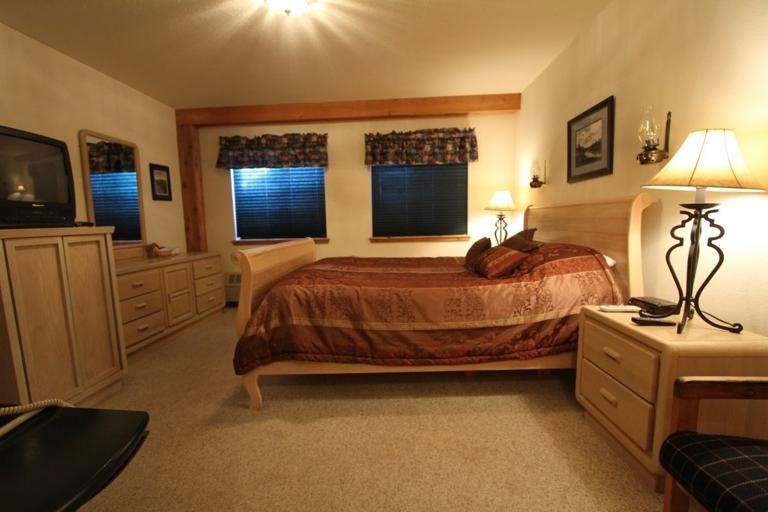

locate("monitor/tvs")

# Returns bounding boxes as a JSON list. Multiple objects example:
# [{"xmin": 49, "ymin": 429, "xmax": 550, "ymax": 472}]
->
[{"xmin": 0, "ymin": 125, "xmax": 76, "ymax": 229}]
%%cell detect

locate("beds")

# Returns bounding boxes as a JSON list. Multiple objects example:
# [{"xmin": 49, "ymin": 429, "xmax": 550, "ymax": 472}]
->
[{"xmin": 227, "ymin": 190, "xmax": 657, "ymax": 411}]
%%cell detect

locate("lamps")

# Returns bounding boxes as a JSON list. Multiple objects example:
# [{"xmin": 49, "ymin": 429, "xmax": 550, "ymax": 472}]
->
[
  {"xmin": 624, "ymin": 124, "xmax": 768, "ymax": 341},
  {"xmin": 527, "ymin": 157, "xmax": 547, "ymax": 190},
  {"xmin": 481, "ymin": 190, "xmax": 516, "ymax": 246},
  {"xmin": 632, "ymin": 105, "xmax": 672, "ymax": 165}
]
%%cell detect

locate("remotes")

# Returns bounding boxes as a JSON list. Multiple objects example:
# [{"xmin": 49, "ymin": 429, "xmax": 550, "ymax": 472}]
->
[
  {"xmin": 600, "ymin": 305, "xmax": 641, "ymax": 312},
  {"xmin": 631, "ymin": 317, "xmax": 676, "ymax": 325},
  {"xmin": 74, "ymin": 221, "xmax": 93, "ymax": 226}
]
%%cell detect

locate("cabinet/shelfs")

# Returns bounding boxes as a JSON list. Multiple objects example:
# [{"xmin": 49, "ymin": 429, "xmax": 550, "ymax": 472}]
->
[
  {"xmin": 111, "ymin": 258, "xmax": 167, "ymax": 352},
  {"xmin": 156, "ymin": 252, "xmax": 197, "ymax": 329},
  {"xmin": 0, "ymin": 223, "xmax": 130, "ymax": 413},
  {"xmin": 186, "ymin": 248, "xmax": 226, "ymax": 317}
]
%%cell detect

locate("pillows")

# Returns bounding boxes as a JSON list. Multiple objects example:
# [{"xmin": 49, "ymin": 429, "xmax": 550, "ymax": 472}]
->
[
  {"xmin": 464, "ymin": 236, "xmax": 490, "ymax": 264},
  {"xmin": 515, "ymin": 228, "xmax": 539, "ymax": 242},
  {"xmin": 463, "ymin": 244, "xmax": 530, "ymax": 281},
  {"xmin": 498, "ymin": 234, "xmax": 544, "ymax": 252}
]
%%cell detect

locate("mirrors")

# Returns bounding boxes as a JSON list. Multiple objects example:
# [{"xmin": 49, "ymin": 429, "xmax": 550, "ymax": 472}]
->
[{"xmin": 76, "ymin": 128, "xmax": 145, "ymax": 245}]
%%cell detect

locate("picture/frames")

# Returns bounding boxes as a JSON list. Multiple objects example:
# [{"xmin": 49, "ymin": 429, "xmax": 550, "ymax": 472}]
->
[
  {"xmin": 147, "ymin": 160, "xmax": 173, "ymax": 201},
  {"xmin": 560, "ymin": 96, "xmax": 615, "ymax": 183}
]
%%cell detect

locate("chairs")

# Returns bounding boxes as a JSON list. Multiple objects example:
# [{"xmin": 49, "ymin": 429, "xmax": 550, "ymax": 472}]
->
[{"xmin": 658, "ymin": 375, "xmax": 768, "ymax": 512}]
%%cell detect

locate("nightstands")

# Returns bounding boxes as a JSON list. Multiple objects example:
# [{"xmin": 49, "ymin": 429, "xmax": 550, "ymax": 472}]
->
[{"xmin": 572, "ymin": 302, "xmax": 768, "ymax": 494}]
